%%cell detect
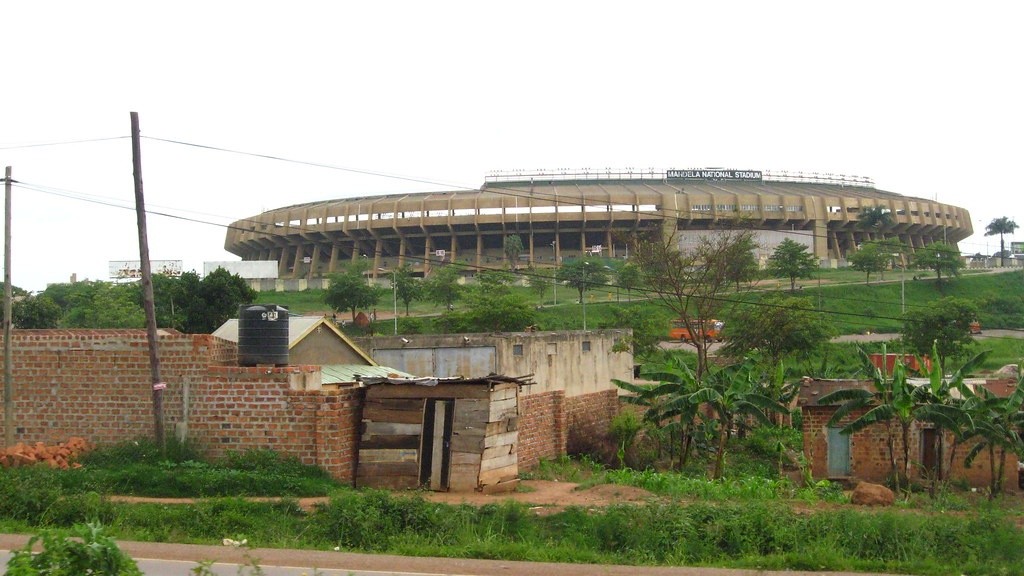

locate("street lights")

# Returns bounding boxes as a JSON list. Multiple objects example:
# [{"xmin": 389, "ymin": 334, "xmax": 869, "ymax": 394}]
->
[
  {"xmin": 363, "ymin": 254, "xmax": 370, "ymax": 287},
  {"xmin": 582, "ymin": 262, "xmax": 589, "ymax": 333},
  {"xmin": 378, "ymin": 267, "xmax": 398, "ymax": 336},
  {"xmin": 818, "ymin": 259, "xmax": 824, "ymax": 323},
  {"xmin": 550, "ymin": 244, "xmax": 556, "ymax": 306}
]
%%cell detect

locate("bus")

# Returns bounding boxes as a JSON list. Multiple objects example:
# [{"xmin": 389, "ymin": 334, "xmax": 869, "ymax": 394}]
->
[{"xmin": 669, "ymin": 319, "xmax": 726, "ymax": 342}]
